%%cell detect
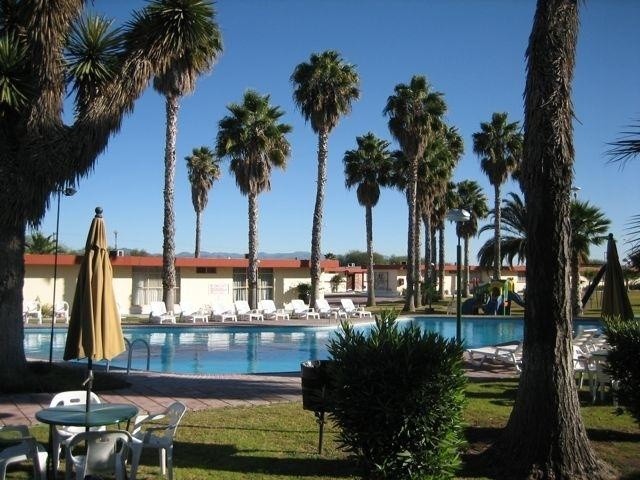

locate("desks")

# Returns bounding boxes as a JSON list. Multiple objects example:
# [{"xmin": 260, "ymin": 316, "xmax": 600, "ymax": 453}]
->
[{"xmin": 33, "ymin": 400, "xmax": 140, "ymax": 480}]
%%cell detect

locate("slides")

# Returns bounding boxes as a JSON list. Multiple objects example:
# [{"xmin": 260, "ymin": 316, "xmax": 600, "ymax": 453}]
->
[{"xmin": 462, "ymin": 292, "xmax": 525, "ymax": 314}]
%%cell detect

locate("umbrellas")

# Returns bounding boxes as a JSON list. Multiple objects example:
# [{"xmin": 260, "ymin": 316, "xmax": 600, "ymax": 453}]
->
[
  {"xmin": 601, "ymin": 233, "xmax": 635, "ymax": 324},
  {"xmin": 63, "ymin": 206, "xmax": 126, "ymax": 452}
]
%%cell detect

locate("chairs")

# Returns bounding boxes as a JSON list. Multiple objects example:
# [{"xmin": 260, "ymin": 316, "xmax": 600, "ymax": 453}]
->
[
  {"xmin": 145, "ymin": 331, "xmax": 330, "ymax": 350},
  {"xmin": 48, "ymin": 388, "xmax": 107, "ymax": 479},
  {"xmin": 50, "ymin": 295, "xmax": 72, "ymax": 328},
  {"xmin": 0, "ymin": 414, "xmax": 51, "ymax": 480},
  {"xmin": 22, "ymin": 297, "xmax": 46, "ymax": 327},
  {"xmin": 146, "ymin": 292, "xmax": 377, "ymax": 328},
  {"xmin": 466, "ymin": 326, "xmax": 618, "ymax": 406},
  {"xmin": 64, "ymin": 427, "xmax": 131, "ymax": 479},
  {"xmin": 128, "ymin": 399, "xmax": 191, "ymax": 479}
]
